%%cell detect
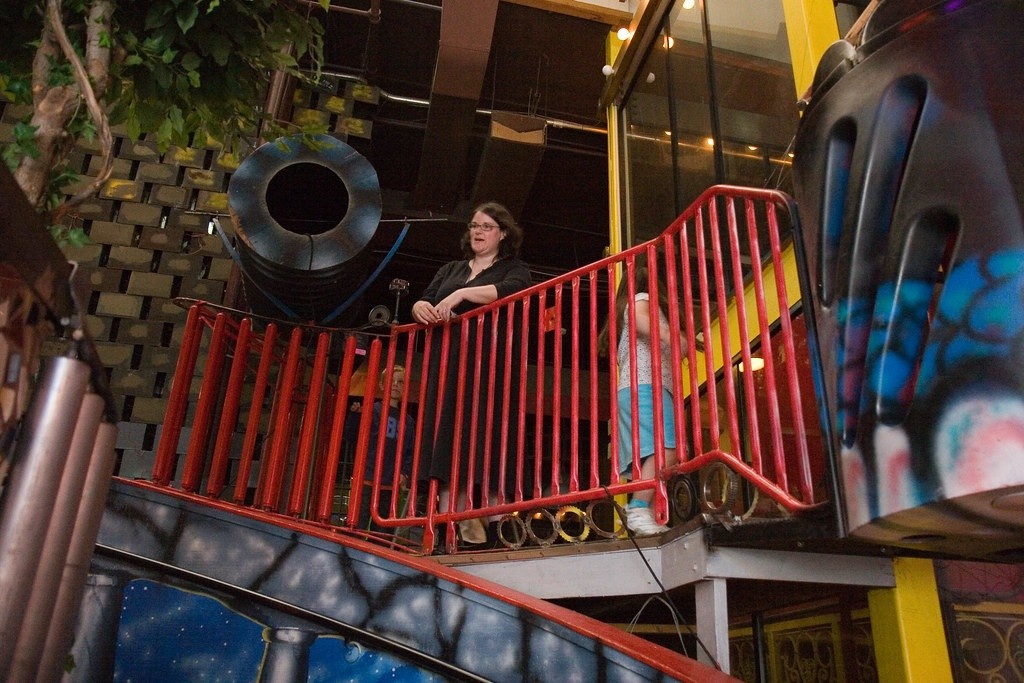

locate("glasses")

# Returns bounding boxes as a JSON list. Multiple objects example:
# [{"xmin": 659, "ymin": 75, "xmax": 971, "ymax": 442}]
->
[{"xmin": 467, "ymin": 221, "xmax": 501, "ymax": 232}]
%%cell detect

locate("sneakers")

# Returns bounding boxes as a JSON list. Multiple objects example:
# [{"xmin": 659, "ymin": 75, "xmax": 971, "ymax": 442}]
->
[{"xmin": 617, "ymin": 504, "xmax": 671, "ymax": 535}]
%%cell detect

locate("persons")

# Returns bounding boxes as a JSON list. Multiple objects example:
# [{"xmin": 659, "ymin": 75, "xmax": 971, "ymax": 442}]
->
[
  {"xmin": 412, "ymin": 203, "xmax": 533, "ymax": 549},
  {"xmin": 347, "ymin": 366, "xmax": 414, "ymax": 542},
  {"xmin": 599, "ymin": 266, "xmax": 704, "ymax": 536}
]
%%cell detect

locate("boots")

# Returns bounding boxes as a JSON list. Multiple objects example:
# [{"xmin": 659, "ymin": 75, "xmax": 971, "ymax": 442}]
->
[
  {"xmin": 488, "ymin": 521, "xmax": 531, "ymax": 546},
  {"xmin": 433, "ymin": 522, "xmax": 459, "ymax": 554}
]
